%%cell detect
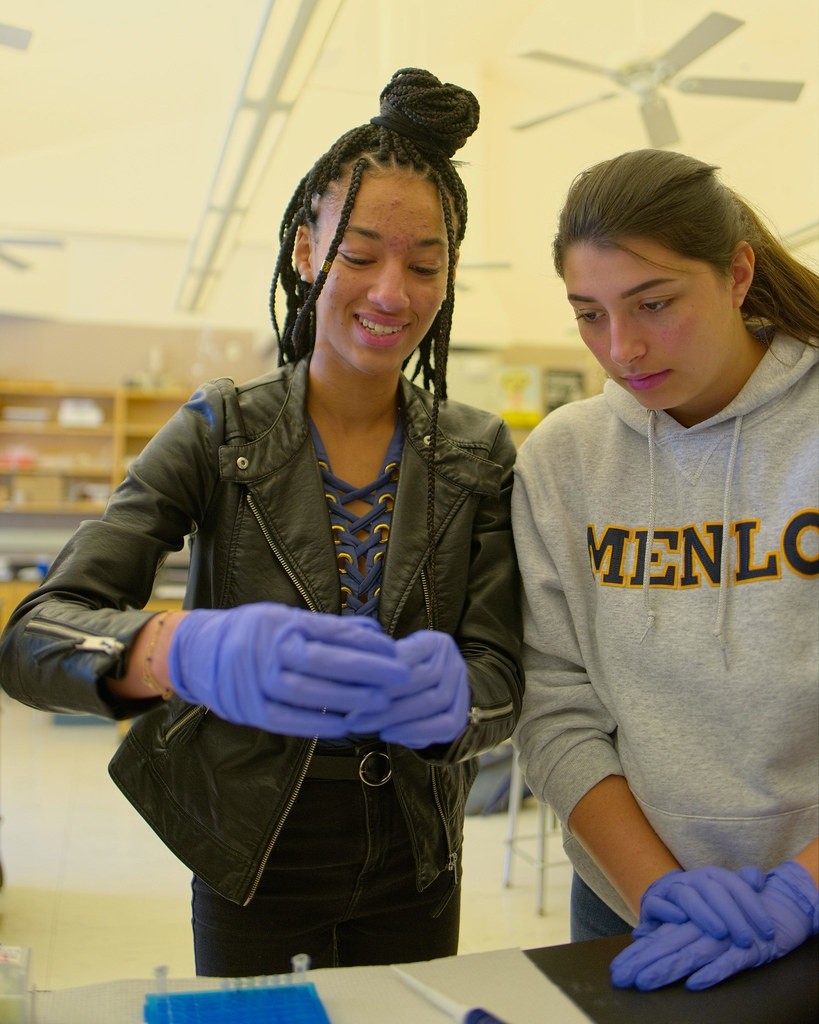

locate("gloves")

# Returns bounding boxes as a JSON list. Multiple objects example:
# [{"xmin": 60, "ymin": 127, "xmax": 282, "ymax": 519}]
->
[
  {"xmin": 167, "ymin": 602, "xmax": 470, "ymax": 750},
  {"xmin": 609, "ymin": 860, "xmax": 819, "ymax": 990}
]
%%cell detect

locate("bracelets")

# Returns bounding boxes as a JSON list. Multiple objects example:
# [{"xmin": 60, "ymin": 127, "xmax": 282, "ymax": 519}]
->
[{"xmin": 143, "ymin": 612, "xmax": 177, "ymax": 702}]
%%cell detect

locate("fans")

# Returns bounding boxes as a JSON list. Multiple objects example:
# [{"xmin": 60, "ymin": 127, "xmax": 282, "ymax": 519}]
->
[{"xmin": 513, "ymin": 11, "xmax": 804, "ymax": 148}]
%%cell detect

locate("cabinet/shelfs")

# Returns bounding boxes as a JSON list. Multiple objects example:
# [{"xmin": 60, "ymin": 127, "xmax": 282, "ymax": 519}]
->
[{"xmin": 0, "ymin": 381, "xmax": 193, "ymax": 634}]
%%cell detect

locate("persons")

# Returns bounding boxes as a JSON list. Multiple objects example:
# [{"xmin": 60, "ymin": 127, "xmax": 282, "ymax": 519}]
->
[
  {"xmin": 506, "ymin": 148, "xmax": 819, "ymax": 989},
  {"xmin": 0, "ymin": 67, "xmax": 530, "ymax": 978}
]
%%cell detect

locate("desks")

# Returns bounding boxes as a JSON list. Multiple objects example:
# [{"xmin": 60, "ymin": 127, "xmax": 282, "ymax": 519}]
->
[{"xmin": 13, "ymin": 920, "xmax": 818, "ymax": 1023}]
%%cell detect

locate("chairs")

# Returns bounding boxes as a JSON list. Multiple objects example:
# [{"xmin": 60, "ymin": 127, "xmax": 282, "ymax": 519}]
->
[{"xmin": 504, "ymin": 742, "xmax": 574, "ymax": 921}]
